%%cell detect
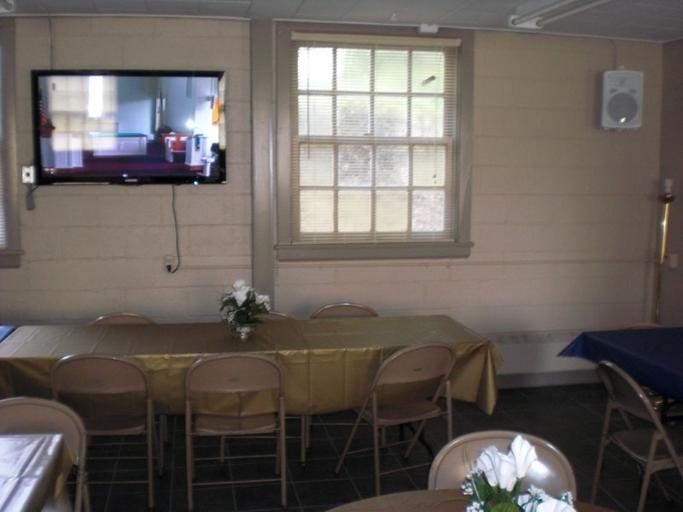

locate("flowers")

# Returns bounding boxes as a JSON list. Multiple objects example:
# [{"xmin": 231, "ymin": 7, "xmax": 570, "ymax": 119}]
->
[{"xmin": 217, "ymin": 280, "xmax": 273, "ymax": 340}]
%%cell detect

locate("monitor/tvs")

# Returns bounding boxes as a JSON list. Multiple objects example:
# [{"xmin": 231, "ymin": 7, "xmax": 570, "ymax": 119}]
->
[{"xmin": 29, "ymin": 67, "xmax": 228, "ymax": 185}]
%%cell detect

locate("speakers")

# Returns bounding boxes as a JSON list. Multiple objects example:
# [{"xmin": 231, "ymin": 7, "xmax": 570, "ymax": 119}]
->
[{"xmin": 600, "ymin": 68, "xmax": 644, "ymax": 130}]
